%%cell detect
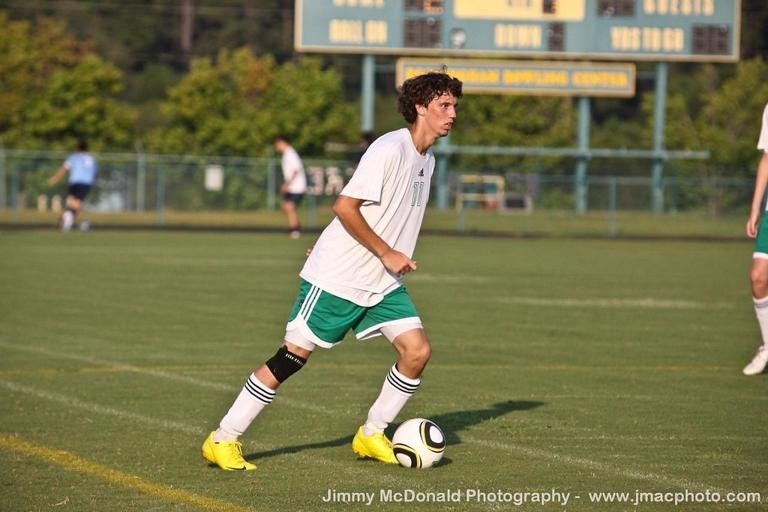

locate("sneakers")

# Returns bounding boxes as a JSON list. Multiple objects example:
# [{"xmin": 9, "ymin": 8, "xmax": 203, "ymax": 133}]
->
[
  {"xmin": 352, "ymin": 425, "xmax": 399, "ymax": 464},
  {"xmin": 742, "ymin": 346, "xmax": 768, "ymax": 375},
  {"xmin": 202, "ymin": 430, "xmax": 257, "ymax": 471}
]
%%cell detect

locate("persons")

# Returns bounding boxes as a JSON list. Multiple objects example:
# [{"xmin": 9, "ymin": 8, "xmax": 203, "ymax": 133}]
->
[
  {"xmin": 273, "ymin": 136, "xmax": 308, "ymax": 239},
  {"xmin": 201, "ymin": 72, "xmax": 463, "ymax": 473},
  {"xmin": 741, "ymin": 104, "xmax": 768, "ymax": 377},
  {"xmin": 46, "ymin": 138, "xmax": 98, "ymax": 231},
  {"xmin": 353, "ymin": 130, "xmax": 377, "ymax": 167}
]
